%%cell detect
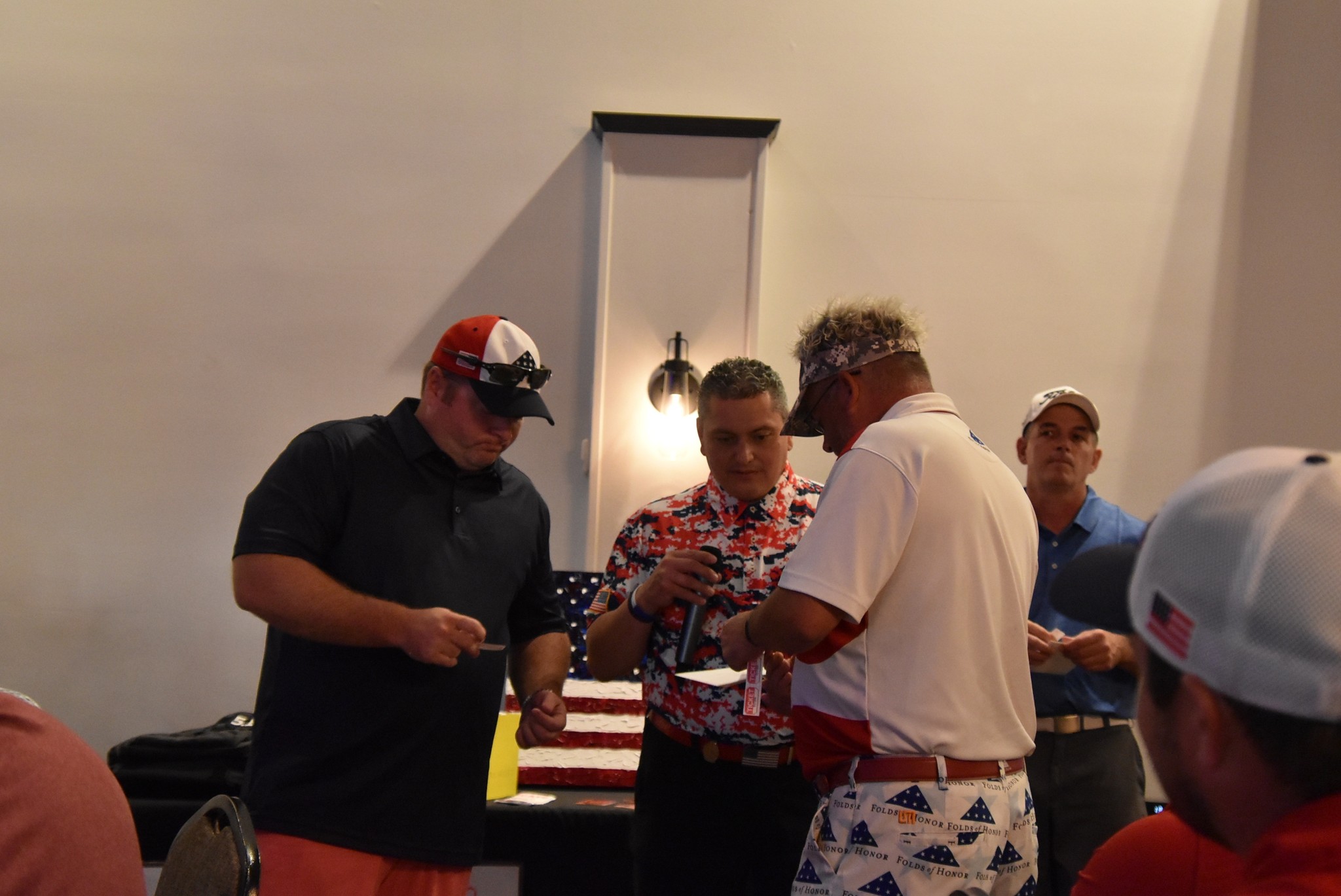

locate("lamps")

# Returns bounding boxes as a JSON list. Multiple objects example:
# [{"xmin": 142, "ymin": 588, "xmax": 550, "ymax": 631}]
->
[{"xmin": 650, "ymin": 330, "xmax": 699, "ymax": 423}]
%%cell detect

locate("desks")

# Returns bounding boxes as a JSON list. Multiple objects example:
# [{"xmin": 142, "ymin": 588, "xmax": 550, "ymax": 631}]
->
[{"xmin": 488, "ymin": 789, "xmax": 635, "ymax": 862}]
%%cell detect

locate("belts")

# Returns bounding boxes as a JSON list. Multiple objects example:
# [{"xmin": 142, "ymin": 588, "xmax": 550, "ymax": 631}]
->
[
  {"xmin": 1036, "ymin": 714, "xmax": 1135, "ymax": 733},
  {"xmin": 645, "ymin": 709, "xmax": 796, "ymax": 768},
  {"xmin": 811, "ymin": 753, "xmax": 1025, "ymax": 801}
]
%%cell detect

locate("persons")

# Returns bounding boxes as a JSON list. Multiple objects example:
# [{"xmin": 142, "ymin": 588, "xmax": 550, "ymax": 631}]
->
[
  {"xmin": 0, "ymin": 691, "xmax": 148, "ymax": 896},
  {"xmin": 231, "ymin": 316, "xmax": 572, "ymax": 896},
  {"xmin": 720, "ymin": 305, "xmax": 1041, "ymax": 896},
  {"xmin": 1016, "ymin": 386, "xmax": 1148, "ymax": 896},
  {"xmin": 586, "ymin": 358, "xmax": 827, "ymax": 896},
  {"xmin": 1070, "ymin": 447, "xmax": 1341, "ymax": 896}
]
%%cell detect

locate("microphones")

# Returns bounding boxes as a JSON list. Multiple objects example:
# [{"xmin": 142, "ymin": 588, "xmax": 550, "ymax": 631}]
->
[{"xmin": 675, "ymin": 546, "xmax": 723, "ymax": 664}]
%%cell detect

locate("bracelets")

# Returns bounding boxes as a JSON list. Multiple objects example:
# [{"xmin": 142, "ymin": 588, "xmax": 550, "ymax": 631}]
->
[
  {"xmin": 628, "ymin": 584, "xmax": 655, "ymax": 623},
  {"xmin": 520, "ymin": 688, "xmax": 553, "ymax": 714},
  {"xmin": 744, "ymin": 619, "xmax": 757, "ymax": 646}
]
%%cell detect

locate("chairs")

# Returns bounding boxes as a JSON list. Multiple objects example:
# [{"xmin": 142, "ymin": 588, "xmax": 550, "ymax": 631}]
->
[{"xmin": 152, "ymin": 795, "xmax": 262, "ymax": 896}]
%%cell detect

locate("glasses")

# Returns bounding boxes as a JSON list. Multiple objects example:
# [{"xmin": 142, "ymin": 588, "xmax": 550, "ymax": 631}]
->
[{"xmin": 440, "ymin": 346, "xmax": 552, "ymax": 390}]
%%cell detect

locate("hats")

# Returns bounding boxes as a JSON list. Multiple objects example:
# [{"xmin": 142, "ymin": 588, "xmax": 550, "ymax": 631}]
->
[
  {"xmin": 780, "ymin": 339, "xmax": 921, "ymax": 437},
  {"xmin": 431, "ymin": 315, "xmax": 555, "ymax": 426},
  {"xmin": 1019, "ymin": 386, "xmax": 1101, "ymax": 445},
  {"xmin": 1128, "ymin": 445, "xmax": 1341, "ymax": 722}
]
